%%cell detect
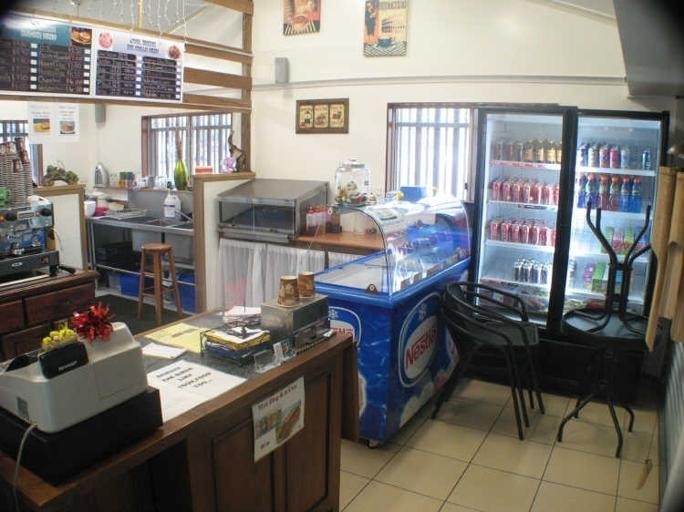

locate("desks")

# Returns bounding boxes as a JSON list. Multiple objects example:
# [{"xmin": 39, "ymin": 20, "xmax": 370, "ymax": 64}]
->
[
  {"xmin": 556, "ymin": 336, "xmax": 660, "ymax": 456},
  {"xmin": 0, "ymin": 267, "xmax": 103, "ymax": 362},
  {"xmin": 564, "ymin": 202, "xmax": 664, "ymax": 340}
]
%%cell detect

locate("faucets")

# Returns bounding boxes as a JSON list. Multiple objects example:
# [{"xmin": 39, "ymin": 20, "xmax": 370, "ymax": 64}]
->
[{"xmin": 174, "ymin": 210, "xmax": 192, "ymax": 223}]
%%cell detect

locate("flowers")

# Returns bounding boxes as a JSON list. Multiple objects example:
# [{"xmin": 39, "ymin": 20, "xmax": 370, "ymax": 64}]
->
[{"xmin": 174, "ymin": 126, "xmax": 189, "ymax": 189}]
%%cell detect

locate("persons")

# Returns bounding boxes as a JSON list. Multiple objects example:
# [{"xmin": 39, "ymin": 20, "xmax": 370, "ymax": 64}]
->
[{"xmin": 366, "ymin": 2, "xmax": 380, "ymax": 44}]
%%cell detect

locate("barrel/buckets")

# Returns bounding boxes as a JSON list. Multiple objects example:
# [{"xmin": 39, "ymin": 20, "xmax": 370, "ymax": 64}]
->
[{"xmin": 163, "ymin": 188, "xmax": 182, "ymax": 218}]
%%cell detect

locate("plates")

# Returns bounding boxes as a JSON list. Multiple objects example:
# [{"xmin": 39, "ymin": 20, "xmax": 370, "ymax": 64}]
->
[
  {"xmin": 33, "ymin": 122, "xmax": 50, "ymax": 133},
  {"xmin": 374, "ymin": 43, "xmax": 397, "ymax": 51},
  {"xmin": 60, "ymin": 123, "xmax": 75, "ymax": 134}
]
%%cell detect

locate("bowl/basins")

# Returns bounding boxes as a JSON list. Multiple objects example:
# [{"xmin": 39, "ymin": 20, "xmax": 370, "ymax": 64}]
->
[
  {"xmin": 379, "ymin": 36, "xmax": 391, "ymax": 47},
  {"xmin": 83, "ymin": 199, "xmax": 97, "ymax": 217},
  {"xmin": 290, "ymin": 15, "xmax": 311, "ymax": 30}
]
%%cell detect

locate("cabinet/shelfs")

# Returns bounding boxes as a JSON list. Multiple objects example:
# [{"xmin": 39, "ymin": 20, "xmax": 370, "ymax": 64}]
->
[{"xmin": 90, "ymin": 216, "xmax": 195, "ymax": 316}]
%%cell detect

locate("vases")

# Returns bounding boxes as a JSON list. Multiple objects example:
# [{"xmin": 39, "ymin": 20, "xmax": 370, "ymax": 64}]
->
[{"xmin": 174, "ymin": 159, "xmax": 188, "ymax": 188}]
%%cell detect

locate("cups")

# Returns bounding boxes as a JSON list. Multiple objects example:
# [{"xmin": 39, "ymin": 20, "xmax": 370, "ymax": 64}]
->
[
  {"xmin": 0, "ymin": 135, "xmax": 33, "ymax": 207},
  {"xmin": 276, "ymin": 270, "xmax": 317, "ymax": 308}
]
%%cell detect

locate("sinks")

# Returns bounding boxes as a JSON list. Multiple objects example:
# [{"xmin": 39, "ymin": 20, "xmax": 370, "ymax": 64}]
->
[
  {"xmin": 131, "ymin": 218, "xmax": 164, "ymax": 252},
  {"xmin": 164, "ymin": 220, "xmax": 195, "ymax": 260}
]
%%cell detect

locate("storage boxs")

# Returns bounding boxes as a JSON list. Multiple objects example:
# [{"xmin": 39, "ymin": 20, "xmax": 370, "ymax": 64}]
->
[
  {"xmin": 178, "ymin": 274, "xmax": 195, "ymax": 314},
  {"xmin": 119, "ymin": 276, "xmax": 141, "ymax": 297}
]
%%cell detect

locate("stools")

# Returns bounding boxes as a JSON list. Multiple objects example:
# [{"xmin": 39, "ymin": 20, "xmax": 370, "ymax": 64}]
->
[{"xmin": 137, "ymin": 244, "xmax": 181, "ymax": 324}]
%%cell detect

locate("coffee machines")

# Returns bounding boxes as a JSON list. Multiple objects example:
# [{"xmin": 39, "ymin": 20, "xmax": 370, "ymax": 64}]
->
[{"xmin": 0, "ymin": 197, "xmax": 61, "ymax": 287}]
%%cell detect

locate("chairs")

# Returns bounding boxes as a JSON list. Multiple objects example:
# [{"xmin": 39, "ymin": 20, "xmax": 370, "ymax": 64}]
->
[{"xmin": 432, "ymin": 281, "xmax": 546, "ymax": 439}]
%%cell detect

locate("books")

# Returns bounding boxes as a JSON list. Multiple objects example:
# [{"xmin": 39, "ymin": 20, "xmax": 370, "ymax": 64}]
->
[
  {"xmin": 206, "ymin": 305, "xmax": 271, "ymax": 361},
  {"xmin": 140, "ymin": 342, "xmax": 188, "ymax": 359}
]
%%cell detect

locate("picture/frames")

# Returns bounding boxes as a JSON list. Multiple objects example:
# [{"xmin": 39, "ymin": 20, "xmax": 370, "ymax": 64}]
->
[
  {"xmin": 30, "ymin": 184, "xmax": 89, "ymax": 273},
  {"xmin": 294, "ymin": 98, "xmax": 351, "ymax": 135}
]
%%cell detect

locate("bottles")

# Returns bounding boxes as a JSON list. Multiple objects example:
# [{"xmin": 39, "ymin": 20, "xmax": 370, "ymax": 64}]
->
[
  {"xmin": 572, "ymin": 173, "xmax": 644, "ymax": 215},
  {"xmin": 305, "ymin": 204, "xmax": 334, "ymax": 236},
  {"xmin": 521, "ymin": 140, "xmax": 565, "ymax": 165}
]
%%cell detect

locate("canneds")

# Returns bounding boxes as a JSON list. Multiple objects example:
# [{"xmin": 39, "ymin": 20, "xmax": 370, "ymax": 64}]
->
[
  {"xmin": 513, "ymin": 257, "xmax": 552, "ymax": 285},
  {"xmin": 489, "ymin": 217, "xmax": 558, "ymax": 245},
  {"xmin": 492, "ymin": 177, "xmax": 560, "ymax": 204},
  {"xmin": 576, "ymin": 140, "xmax": 653, "ymax": 170}
]
